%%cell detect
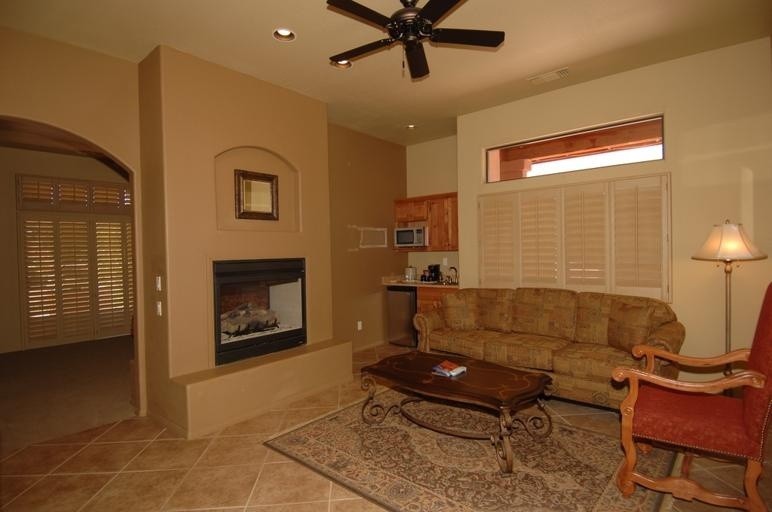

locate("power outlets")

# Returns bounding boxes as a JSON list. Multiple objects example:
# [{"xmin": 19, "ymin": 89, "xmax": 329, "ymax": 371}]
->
[
  {"xmin": 157, "ymin": 301, "xmax": 162, "ymax": 316},
  {"xmin": 156, "ymin": 276, "xmax": 162, "ymax": 292}
]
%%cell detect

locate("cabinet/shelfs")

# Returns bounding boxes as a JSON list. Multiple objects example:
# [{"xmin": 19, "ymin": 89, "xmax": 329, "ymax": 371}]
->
[
  {"xmin": 383, "ymin": 274, "xmax": 459, "ymax": 347},
  {"xmin": 394, "ymin": 191, "xmax": 459, "ymax": 252}
]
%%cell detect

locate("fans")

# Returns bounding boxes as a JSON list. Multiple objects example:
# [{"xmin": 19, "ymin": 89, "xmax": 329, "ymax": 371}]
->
[{"xmin": 327, "ymin": 0, "xmax": 505, "ymax": 80}]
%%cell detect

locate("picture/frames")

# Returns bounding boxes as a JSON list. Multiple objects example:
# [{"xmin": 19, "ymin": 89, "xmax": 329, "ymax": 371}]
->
[{"xmin": 234, "ymin": 169, "xmax": 279, "ymax": 220}]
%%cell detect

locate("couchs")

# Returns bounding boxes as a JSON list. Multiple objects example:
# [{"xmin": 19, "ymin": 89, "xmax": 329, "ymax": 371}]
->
[
  {"xmin": 413, "ymin": 287, "xmax": 685, "ymax": 410},
  {"xmin": 612, "ymin": 280, "xmax": 772, "ymax": 511}
]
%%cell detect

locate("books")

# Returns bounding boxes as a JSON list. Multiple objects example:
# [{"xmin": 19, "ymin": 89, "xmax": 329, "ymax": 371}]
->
[{"xmin": 434, "ymin": 357, "xmax": 465, "ymax": 376}]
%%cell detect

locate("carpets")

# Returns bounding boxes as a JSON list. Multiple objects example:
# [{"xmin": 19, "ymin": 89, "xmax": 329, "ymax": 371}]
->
[{"xmin": 262, "ymin": 388, "xmax": 684, "ymax": 512}]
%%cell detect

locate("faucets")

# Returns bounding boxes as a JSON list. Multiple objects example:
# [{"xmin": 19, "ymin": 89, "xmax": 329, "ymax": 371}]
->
[{"xmin": 449, "ymin": 267, "xmax": 457, "ymax": 285}]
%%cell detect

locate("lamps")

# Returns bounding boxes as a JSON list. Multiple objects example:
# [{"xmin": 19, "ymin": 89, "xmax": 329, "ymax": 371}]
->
[{"xmin": 691, "ymin": 219, "xmax": 767, "ymax": 462}]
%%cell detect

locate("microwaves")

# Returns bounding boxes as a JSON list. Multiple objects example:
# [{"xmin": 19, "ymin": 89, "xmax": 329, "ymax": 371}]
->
[{"xmin": 394, "ymin": 227, "xmax": 424, "ymax": 246}]
